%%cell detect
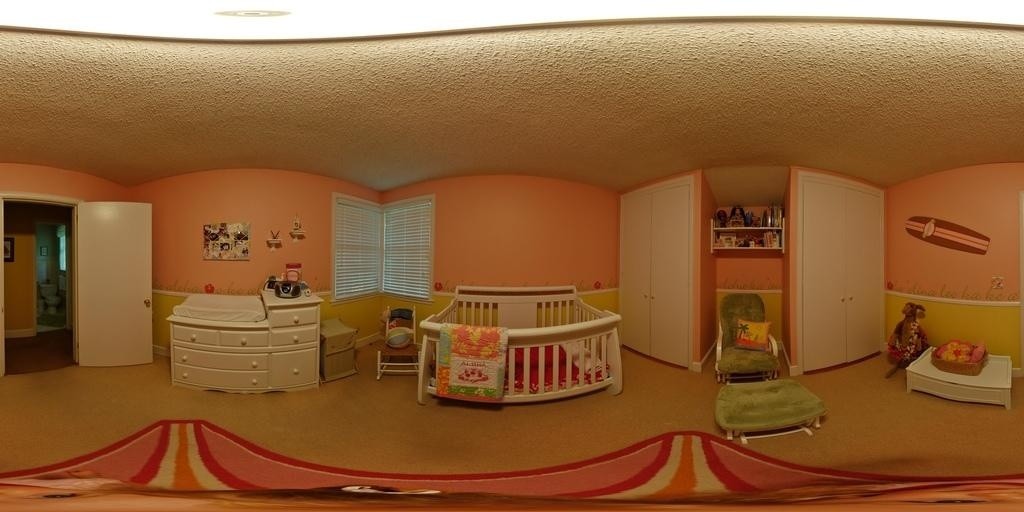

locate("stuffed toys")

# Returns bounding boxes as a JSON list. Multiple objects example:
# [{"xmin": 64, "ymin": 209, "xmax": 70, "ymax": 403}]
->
[{"xmin": 879, "ymin": 302, "xmax": 929, "ymax": 378}]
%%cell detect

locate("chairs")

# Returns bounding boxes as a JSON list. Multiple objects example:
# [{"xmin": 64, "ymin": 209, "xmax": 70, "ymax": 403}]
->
[
  {"xmin": 375, "ymin": 306, "xmax": 422, "ymax": 379},
  {"xmin": 716, "ymin": 292, "xmax": 783, "ymax": 383}
]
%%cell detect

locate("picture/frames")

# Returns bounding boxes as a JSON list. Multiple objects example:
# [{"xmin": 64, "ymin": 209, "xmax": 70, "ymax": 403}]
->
[
  {"xmin": 201, "ymin": 218, "xmax": 251, "ymax": 262},
  {"xmin": 40, "ymin": 246, "xmax": 49, "ymax": 256},
  {"xmin": 3, "ymin": 237, "xmax": 14, "ymax": 263}
]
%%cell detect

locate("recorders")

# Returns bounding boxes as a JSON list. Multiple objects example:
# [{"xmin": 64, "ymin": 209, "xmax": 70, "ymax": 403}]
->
[{"xmin": 275, "ymin": 281, "xmax": 301, "ymax": 298}]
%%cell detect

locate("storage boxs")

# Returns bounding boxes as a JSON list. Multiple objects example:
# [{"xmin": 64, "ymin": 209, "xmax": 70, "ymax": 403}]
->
[{"xmin": 320, "ymin": 314, "xmax": 360, "ymax": 384}]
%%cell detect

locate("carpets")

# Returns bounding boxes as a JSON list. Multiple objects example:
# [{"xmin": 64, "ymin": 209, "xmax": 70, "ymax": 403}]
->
[{"xmin": 0, "ymin": 416, "xmax": 1024, "ymax": 512}]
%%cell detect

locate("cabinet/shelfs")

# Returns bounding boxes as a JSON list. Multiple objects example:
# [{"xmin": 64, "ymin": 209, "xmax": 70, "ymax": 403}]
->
[
  {"xmin": 616, "ymin": 167, "xmax": 888, "ymax": 378},
  {"xmin": 167, "ymin": 289, "xmax": 321, "ymax": 393}
]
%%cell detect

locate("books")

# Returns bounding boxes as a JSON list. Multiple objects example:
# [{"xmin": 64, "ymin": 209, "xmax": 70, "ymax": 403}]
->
[{"xmin": 759, "ymin": 205, "xmax": 782, "ymax": 248}]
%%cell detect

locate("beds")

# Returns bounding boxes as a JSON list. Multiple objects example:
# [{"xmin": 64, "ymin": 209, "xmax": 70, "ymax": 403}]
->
[
  {"xmin": 415, "ymin": 282, "xmax": 624, "ymax": 410},
  {"xmin": 905, "ymin": 345, "xmax": 1013, "ymax": 410}
]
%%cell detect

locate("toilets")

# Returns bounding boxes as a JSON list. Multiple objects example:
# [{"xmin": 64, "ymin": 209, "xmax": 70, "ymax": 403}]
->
[{"xmin": 39, "ymin": 283, "xmax": 61, "ymax": 313}]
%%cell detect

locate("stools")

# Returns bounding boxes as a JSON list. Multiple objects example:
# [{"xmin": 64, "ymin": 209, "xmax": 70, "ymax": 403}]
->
[{"xmin": 714, "ymin": 379, "xmax": 830, "ymax": 441}]
